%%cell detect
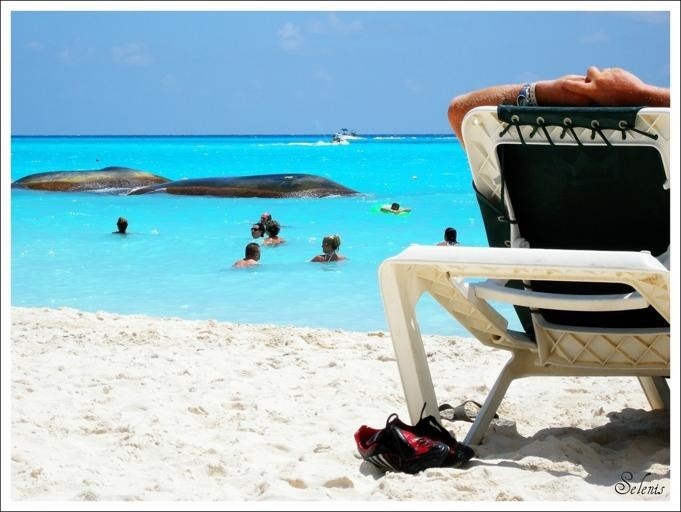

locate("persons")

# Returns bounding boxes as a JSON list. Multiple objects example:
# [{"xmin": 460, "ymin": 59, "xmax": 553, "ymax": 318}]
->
[
  {"xmin": 262, "ymin": 221, "xmax": 284, "ymax": 244},
  {"xmin": 447, "ymin": 66, "xmax": 670, "ymax": 155},
  {"xmin": 381, "ymin": 203, "xmax": 411, "ymax": 213},
  {"xmin": 112, "ymin": 218, "xmax": 131, "ymax": 234},
  {"xmin": 308, "ymin": 235, "xmax": 346, "ymax": 262},
  {"xmin": 251, "ymin": 223, "xmax": 264, "ymax": 244},
  {"xmin": 437, "ymin": 228, "xmax": 464, "ymax": 245},
  {"xmin": 234, "ymin": 243, "xmax": 261, "ymax": 269},
  {"xmin": 259, "ymin": 214, "xmax": 273, "ymax": 224}
]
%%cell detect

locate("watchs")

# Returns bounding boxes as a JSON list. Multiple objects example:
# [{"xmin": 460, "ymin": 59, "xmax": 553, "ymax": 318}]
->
[{"xmin": 517, "ymin": 83, "xmax": 528, "ymax": 106}]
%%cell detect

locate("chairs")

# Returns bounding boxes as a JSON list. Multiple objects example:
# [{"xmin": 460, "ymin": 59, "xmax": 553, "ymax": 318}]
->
[{"xmin": 376, "ymin": 103, "xmax": 672, "ymax": 461}]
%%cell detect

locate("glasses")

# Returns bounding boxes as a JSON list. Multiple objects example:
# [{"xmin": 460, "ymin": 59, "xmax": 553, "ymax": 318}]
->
[{"xmin": 251, "ymin": 228, "xmax": 259, "ymax": 230}]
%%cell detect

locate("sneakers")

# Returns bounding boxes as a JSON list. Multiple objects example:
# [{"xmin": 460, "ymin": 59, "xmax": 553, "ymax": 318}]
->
[{"xmin": 354, "ymin": 415, "xmax": 474, "ymax": 473}]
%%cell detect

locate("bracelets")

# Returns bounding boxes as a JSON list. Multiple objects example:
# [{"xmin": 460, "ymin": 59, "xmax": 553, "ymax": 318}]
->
[{"xmin": 529, "ymin": 81, "xmax": 537, "ymax": 106}]
